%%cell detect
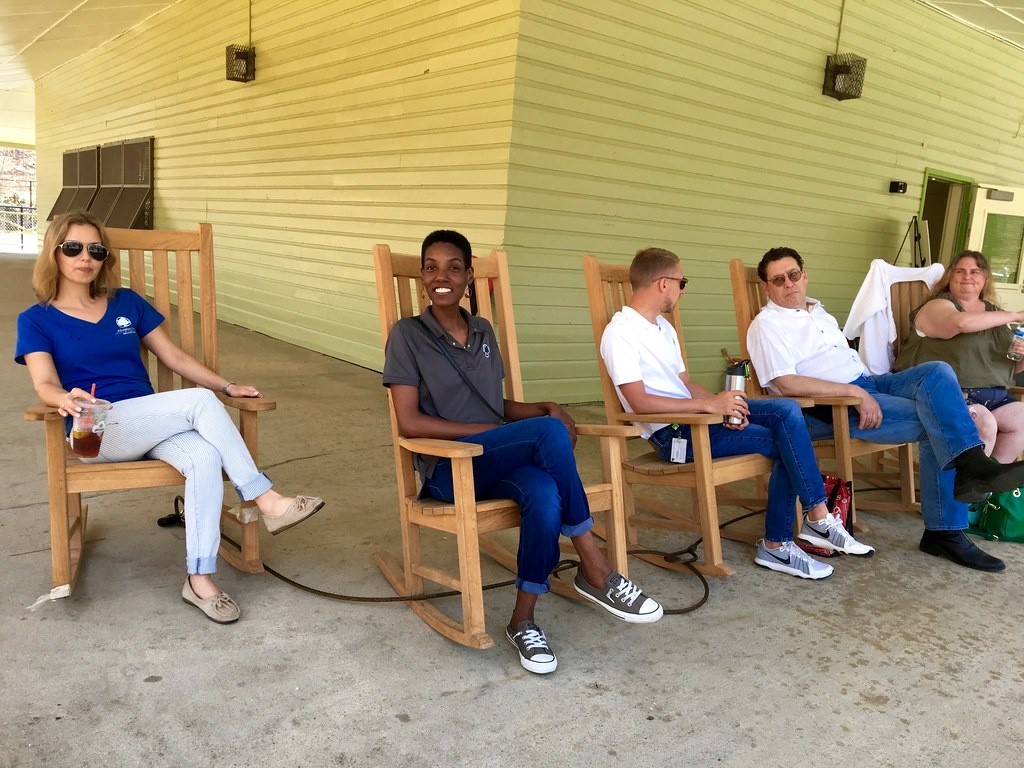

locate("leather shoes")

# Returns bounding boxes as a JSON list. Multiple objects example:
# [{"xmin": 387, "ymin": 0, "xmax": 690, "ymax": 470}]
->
[
  {"xmin": 953, "ymin": 447, "xmax": 1024, "ymax": 502},
  {"xmin": 919, "ymin": 529, "xmax": 1007, "ymax": 572}
]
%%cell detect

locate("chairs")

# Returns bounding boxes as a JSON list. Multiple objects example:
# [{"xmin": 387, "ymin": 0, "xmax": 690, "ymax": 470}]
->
[
  {"xmin": 25, "ymin": 221, "xmax": 278, "ymax": 600},
  {"xmin": 373, "ymin": 244, "xmax": 946, "ymax": 648}
]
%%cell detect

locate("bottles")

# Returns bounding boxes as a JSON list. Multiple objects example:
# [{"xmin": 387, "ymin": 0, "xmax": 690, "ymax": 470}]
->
[{"xmin": 1006, "ymin": 323, "xmax": 1024, "ymax": 361}]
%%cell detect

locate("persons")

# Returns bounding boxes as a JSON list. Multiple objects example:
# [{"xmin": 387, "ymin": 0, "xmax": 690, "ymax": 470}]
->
[
  {"xmin": 599, "ymin": 248, "xmax": 875, "ymax": 580},
  {"xmin": 14, "ymin": 212, "xmax": 325, "ymax": 625},
  {"xmin": 891, "ymin": 250, "xmax": 1024, "ymax": 463},
  {"xmin": 746, "ymin": 247, "xmax": 1024, "ymax": 571},
  {"xmin": 381, "ymin": 230, "xmax": 664, "ymax": 674}
]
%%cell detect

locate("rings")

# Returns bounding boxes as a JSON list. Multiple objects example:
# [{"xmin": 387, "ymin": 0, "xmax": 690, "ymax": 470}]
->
[{"xmin": 62, "ymin": 403, "xmax": 65, "ymax": 408}]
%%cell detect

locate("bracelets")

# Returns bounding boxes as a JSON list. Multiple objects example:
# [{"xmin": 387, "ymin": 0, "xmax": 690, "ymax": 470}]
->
[{"xmin": 223, "ymin": 381, "xmax": 236, "ymax": 394}]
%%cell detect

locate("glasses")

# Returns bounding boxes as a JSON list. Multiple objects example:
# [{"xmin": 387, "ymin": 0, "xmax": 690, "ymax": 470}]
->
[
  {"xmin": 767, "ymin": 270, "xmax": 803, "ymax": 287},
  {"xmin": 652, "ymin": 277, "xmax": 689, "ymax": 290},
  {"xmin": 58, "ymin": 241, "xmax": 110, "ymax": 262}
]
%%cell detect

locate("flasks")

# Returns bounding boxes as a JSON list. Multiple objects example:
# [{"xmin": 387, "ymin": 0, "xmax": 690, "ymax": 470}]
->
[{"xmin": 723, "ymin": 359, "xmax": 750, "ymax": 424}]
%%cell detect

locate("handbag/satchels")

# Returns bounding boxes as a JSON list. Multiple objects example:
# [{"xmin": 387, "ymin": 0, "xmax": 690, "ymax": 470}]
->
[
  {"xmin": 981, "ymin": 487, "xmax": 1024, "ymax": 543},
  {"xmin": 796, "ymin": 473, "xmax": 852, "ymax": 557}
]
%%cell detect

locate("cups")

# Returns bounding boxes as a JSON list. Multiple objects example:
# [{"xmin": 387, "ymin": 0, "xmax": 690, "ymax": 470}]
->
[{"xmin": 71, "ymin": 397, "xmax": 111, "ymax": 458}]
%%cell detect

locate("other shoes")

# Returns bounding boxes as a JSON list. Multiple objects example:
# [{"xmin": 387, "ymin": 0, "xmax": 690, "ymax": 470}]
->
[
  {"xmin": 262, "ymin": 496, "xmax": 325, "ymax": 536},
  {"xmin": 181, "ymin": 575, "xmax": 241, "ymax": 624}
]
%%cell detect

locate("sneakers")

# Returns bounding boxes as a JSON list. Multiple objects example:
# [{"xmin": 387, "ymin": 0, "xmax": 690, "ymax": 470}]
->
[
  {"xmin": 753, "ymin": 538, "xmax": 834, "ymax": 580},
  {"xmin": 505, "ymin": 620, "xmax": 557, "ymax": 674},
  {"xmin": 797, "ymin": 513, "xmax": 874, "ymax": 558},
  {"xmin": 573, "ymin": 565, "xmax": 663, "ymax": 623}
]
